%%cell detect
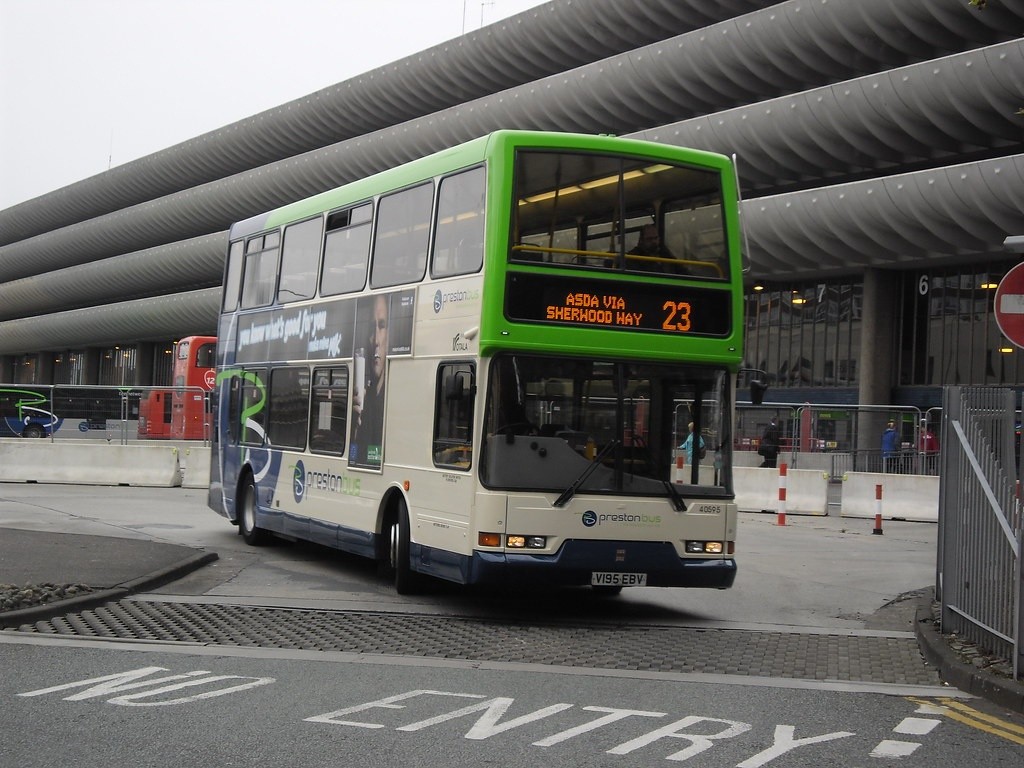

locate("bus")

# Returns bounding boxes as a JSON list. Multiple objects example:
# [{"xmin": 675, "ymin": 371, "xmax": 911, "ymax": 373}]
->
[
  {"xmin": 171, "ymin": 336, "xmax": 221, "ymax": 443},
  {"xmin": 0, "ymin": 384, "xmax": 145, "ymax": 440},
  {"xmin": 206, "ymin": 129, "xmax": 769, "ymax": 612}
]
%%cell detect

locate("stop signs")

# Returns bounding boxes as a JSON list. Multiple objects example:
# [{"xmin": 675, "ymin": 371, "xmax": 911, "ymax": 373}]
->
[{"xmin": 994, "ymin": 261, "xmax": 1024, "ymax": 350}]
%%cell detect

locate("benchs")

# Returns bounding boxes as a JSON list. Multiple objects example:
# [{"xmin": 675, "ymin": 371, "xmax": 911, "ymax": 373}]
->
[{"xmin": 540, "ymin": 423, "xmax": 590, "ymax": 449}]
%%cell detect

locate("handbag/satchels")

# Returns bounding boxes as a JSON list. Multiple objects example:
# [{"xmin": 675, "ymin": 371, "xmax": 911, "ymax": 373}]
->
[
  {"xmin": 758, "ymin": 438, "xmax": 779, "ymax": 456},
  {"xmin": 700, "ymin": 445, "xmax": 706, "ymax": 459}
]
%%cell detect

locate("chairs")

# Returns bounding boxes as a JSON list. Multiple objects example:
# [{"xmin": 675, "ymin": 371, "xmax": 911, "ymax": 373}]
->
[
  {"xmin": 513, "ymin": 242, "xmax": 542, "ymax": 262},
  {"xmin": 571, "ymin": 250, "xmax": 622, "ymax": 266},
  {"xmin": 458, "ymin": 239, "xmax": 485, "ymax": 271}
]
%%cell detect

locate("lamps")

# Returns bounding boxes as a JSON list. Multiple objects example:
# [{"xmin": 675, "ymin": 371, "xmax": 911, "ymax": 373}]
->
[
  {"xmin": 999, "ymin": 333, "xmax": 1013, "ymax": 353},
  {"xmin": 981, "ymin": 273, "xmax": 998, "ymax": 288},
  {"xmin": 791, "ymin": 293, "xmax": 806, "ymax": 304}
]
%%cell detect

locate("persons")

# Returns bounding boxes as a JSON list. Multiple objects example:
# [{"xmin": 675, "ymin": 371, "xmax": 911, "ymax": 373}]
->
[
  {"xmin": 624, "ymin": 224, "xmax": 685, "ymax": 274},
  {"xmin": 352, "ymin": 293, "xmax": 390, "ymax": 467},
  {"xmin": 880, "ymin": 422, "xmax": 898, "ymax": 473},
  {"xmin": 758, "ymin": 416, "xmax": 780, "ymax": 468},
  {"xmin": 677, "ymin": 421, "xmax": 706, "ymax": 485},
  {"xmin": 918, "ymin": 422, "xmax": 937, "ymax": 475}
]
